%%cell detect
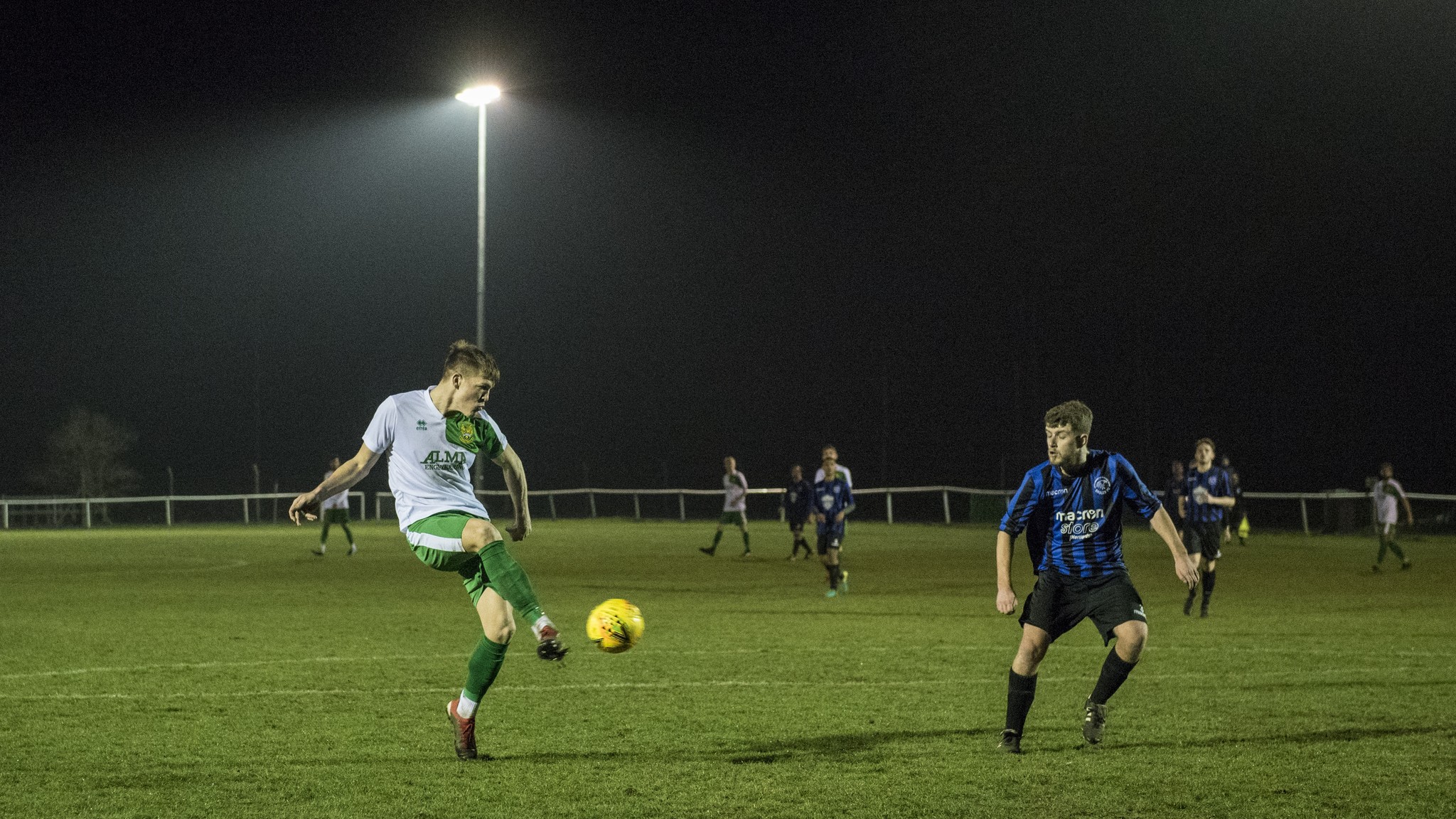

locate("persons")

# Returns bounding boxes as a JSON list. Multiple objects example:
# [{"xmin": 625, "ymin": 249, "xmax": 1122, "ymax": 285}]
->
[
  {"xmin": 1219, "ymin": 453, "xmax": 1246, "ymax": 545},
  {"xmin": 311, "ymin": 456, "xmax": 356, "ymax": 557},
  {"xmin": 814, "ymin": 445, "xmax": 855, "ymax": 555},
  {"xmin": 1159, "ymin": 458, "xmax": 1191, "ymax": 547},
  {"xmin": 1367, "ymin": 463, "xmax": 1416, "ymax": 575},
  {"xmin": 1176, "ymin": 434, "xmax": 1235, "ymax": 624},
  {"xmin": 993, "ymin": 400, "xmax": 1202, "ymax": 753},
  {"xmin": 290, "ymin": 340, "xmax": 571, "ymax": 758},
  {"xmin": 808, "ymin": 455, "xmax": 857, "ymax": 598},
  {"xmin": 775, "ymin": 460, "xmax": 818, "ymax": 563},
  {"xmin": 699, "ymin": 453, "xmax": 753, "ymax": 559}
]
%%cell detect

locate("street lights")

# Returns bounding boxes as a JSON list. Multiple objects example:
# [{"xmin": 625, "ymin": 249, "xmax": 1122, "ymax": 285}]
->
[{"xmin": 456, "ymin": 79, "xmax": 505, "ymax": 508}]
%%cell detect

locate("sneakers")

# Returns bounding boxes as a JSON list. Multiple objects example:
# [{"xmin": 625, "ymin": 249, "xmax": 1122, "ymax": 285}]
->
[
  {"xmin": 1200, "ymin": 604, "xmax": 1209, "ymax": 618},
  {"xmin": 312, "ymin": 549, "xmax": 325, "ymax": 558},
  {"xmin": 805, "ymin": 551, "xmax": 813, "ymax": 560},
  {"xmin": 825, "ymin": 589, "xmax": 837, "ymax": 598},
  {"xmin": 1083, "ymin": 693, "xmax": 1108, "ymax": 743},
  {"xmin": 840, "ymin": 570, "xmax": 849, "ymax": 592},
  {"xmin": 446, "ymin": 699, "xmax": 478, "ymax": 761},
  {"xmin": 700, "ymin": 547, "xmax": 715, "ymax": 556},
  {"xmin": 537, "ymin": 625, "xmax": 570, "ymax": 661},
  {"xmin": 996, "ymin": 729, "xmax": 1022, "ymax": 754},
  {"xmin": 347, "ymin": 547, "xmax": 358, "ymax": 556},
  {"xmin": 743, "ymin": 549, "xmax": 752, "ymax": 557},
  {"xmin": 1183, "ymin": 596, "xmax": 1196, "ymax": 615},
  {"xmin": 785, "ymin": 555, "xmax": 796, "ymax": 561}
]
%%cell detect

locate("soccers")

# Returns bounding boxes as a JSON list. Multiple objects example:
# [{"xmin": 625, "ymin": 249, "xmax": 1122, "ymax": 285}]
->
[{"xmin": 585, "ymin": 598, "xmax": 645, "ymax": 653}]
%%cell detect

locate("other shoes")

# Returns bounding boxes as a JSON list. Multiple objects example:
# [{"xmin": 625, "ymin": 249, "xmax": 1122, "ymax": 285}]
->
[
  {"xmin": 1372, "ymin": 564, "xmax": 1382, "ymax": 570},
  {"xmin": 1402, "ymin": 562, "xmax": 1411, "ymax": 571}
]
